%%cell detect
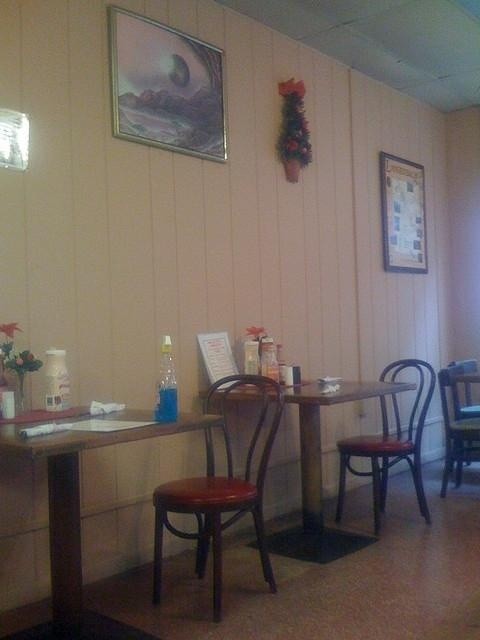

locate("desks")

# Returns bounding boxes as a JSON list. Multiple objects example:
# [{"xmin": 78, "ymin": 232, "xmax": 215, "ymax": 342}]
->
[
  {"xmin": 0, "ymin": 404, "xmax": 224, "ymax": 640},
  {"xmin": 199, "ymin": 376, "xmax": 418, "ymax": 566}
]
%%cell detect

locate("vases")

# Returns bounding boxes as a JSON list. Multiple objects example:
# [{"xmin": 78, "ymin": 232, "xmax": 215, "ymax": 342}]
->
[
  {"xmin": 284, "ymin": 158, "xmax": 300, "ymax": 183},
  {"xmin": 15, "ymin": 392, "xmax": 29, "ymax": 415}
]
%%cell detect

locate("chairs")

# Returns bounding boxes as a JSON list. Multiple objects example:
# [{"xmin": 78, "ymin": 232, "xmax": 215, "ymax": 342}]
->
[
  {"xmin": 438, "ymin": 359, "xmax": 479, "ymax": 500},
  {"xmin": 153, "ymin": 372, "xmax": 287, "ymax": 624},
  {"xmin": 335, "ymin": 359, "xmax": 438, "ymax": 536}
]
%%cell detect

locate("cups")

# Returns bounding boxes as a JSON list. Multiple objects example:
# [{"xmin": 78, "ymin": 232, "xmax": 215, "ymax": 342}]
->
[
  {"xmin": 280, "ymin": 365, "xmax": 301, "ymax": 386},
  {"xmin": 317, "ymin": 377, "xmax": 343, "ymax": 394},
  {"xmin": 0, "ymin": 390, "xmax": 15, "ymax": 419}
]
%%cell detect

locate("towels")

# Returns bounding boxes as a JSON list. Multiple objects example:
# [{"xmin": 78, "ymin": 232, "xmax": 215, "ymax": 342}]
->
[
  {"xmin": 90, "ymin": 401, "xmax": 126, "ymax": 416},
  {"xmin": 20, "ymin": 421, "xmax": 72, "ymax": 437}
]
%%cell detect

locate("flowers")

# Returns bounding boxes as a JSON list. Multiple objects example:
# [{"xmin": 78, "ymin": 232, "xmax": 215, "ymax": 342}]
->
[
  {"xmin": 243, "ymin": 325, "xmax": 269, "ymax": 356},
  {"xmin": 277, "ymin": 78, "xmax": 312, "ymax": 168},
  {"xmin": 0, "ymin": 321, "xmax": 43, "ymax": 395}
]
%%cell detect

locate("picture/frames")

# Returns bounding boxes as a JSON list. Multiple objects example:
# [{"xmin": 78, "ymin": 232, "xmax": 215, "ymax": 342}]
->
[
  {"xmin": 107, "ymin": 4, "xmax": 228, "ymax": 164},
  {"xmin": 379, "ymin": 151, "xmax": 429, "ymax": 274}
]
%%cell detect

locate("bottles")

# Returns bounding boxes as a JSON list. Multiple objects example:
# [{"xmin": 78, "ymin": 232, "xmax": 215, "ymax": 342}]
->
[
  {"xmin": 43, "ymin": 350, "xmax": 72, "ymax": 410},
  {"xmin": 154, "ymin": 334, "xmax": 179, "ymax": 424},
  {"xmin": 244, "ymin": 340, "xmax": 279, "ymax": 387}
]
